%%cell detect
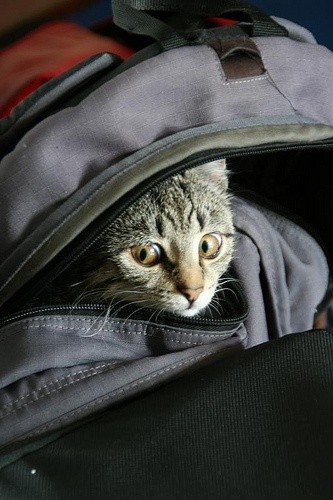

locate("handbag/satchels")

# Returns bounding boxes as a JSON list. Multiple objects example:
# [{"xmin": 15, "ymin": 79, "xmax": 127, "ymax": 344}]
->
[{"xmin": 1, "ymin": 0, "xmax": 331, "ymax": 451}]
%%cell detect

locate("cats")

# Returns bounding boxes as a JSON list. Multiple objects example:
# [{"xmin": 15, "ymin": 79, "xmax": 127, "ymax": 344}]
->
[{"xmin": 69, "ymin": 158, "xmax": 242, "ymax": 338}]
